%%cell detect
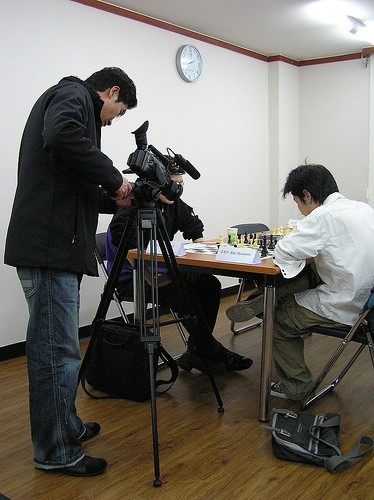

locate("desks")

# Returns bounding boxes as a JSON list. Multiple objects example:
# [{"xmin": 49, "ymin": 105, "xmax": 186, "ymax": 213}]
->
[{"xmin": 127, "ymin": 229, "xmax": 314, "ymax": 422}]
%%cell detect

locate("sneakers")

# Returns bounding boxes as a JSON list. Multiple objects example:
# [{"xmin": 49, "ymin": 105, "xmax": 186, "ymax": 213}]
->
[
  {"xmin": 226, "ymin": 293, "xmax": 278, "ymax": 322},
  {"xmin": 270, "ymin": 382, "xmax": 289, "ymax": 399}
]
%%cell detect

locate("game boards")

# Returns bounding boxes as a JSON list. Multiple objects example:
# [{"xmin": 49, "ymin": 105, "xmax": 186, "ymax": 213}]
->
[
  {"xmin": 255, "ymin": 230, "xmax": 289, "ymax": 241},
  {"xmin": 181, "ymin": 241, "xmax": 275, "ymax": 260}
]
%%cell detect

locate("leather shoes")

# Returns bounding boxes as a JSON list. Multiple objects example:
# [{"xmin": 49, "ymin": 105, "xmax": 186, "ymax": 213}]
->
[
  {"xmin": 79, "ymin": 422, "xmax": 101, "ymax": 442},
  {"xmin": 35, "ymin": 455, "xmax": 108, "ymax": 476},
  {"xmin": 219, "ymin": 345, "xmax": 254, "ymax": 372},
  {"xmin": 178, "ymin": 350, "xmax": 225, "ymax": 374}
]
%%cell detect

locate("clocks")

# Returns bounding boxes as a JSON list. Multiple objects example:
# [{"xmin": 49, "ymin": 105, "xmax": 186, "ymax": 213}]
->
[{"xmin": 177, "ymin": 45, "xmax": 203, "ymax": 82}]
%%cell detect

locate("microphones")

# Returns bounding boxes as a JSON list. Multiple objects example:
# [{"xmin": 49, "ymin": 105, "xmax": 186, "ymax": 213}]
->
[{"xmin": 174, "ymin": 153, "xmax": 200, "ymax": 180}]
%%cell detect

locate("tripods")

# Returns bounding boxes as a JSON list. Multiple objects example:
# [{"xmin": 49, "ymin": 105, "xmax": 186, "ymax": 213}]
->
[{"xmin": 75, "ymin": 185, "xmax": 224, "ymax": 488}]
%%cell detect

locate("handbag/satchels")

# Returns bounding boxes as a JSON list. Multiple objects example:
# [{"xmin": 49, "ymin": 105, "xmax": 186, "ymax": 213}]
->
[
  {"xmin": 81, "ymin": 321, "xmax": 179, "ymax": 402},
  {"xmin": 264, "ymin": 407, "xmax": 341, "ymax": 466}
]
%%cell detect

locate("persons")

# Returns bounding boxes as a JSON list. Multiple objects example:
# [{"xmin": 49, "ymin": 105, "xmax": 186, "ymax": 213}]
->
[
  {"xmin": 4, "ymin": 66, "xmax": 139, "ymax": 480},
  {"xmin": 105, "ymin": 167, "xmax": 254, "ymax": 375},
  {"xmin": 227, "ymin": 157, "xmax": 374, "ymax": 403}
]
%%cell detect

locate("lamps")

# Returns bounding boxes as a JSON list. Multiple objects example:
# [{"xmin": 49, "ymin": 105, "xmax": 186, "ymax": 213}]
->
[{"xmin": 346, "ymin": 15, "xmax": 365, "ymax": 34}]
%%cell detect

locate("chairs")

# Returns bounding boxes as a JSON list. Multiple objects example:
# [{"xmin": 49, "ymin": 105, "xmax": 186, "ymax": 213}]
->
[
  {"xmin": 94, "ymin": 233, "xmax": 187, "ymax": 365},
  {"xmin": 230, "ymin": 223, "xmax": 270, "ymax": 335},
  {"xmin": 301, "ymin": 286, "xmax": 374, "ymax": 411}
]
